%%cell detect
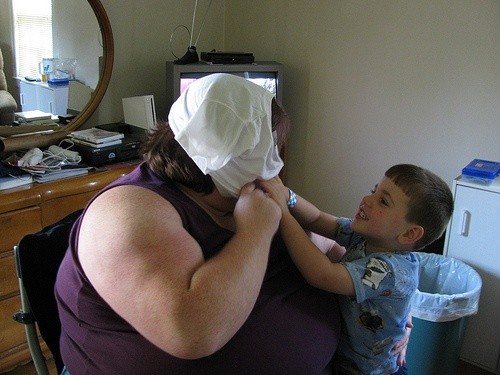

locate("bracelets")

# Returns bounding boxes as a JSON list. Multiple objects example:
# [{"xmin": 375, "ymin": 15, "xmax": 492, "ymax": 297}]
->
[{"xmin": 286, "ymin": 189, "xmax": 296, "ymax": 208}]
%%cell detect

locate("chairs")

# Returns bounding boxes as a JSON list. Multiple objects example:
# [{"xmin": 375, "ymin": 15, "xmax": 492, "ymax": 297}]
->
[{"xmin": 14, "ymin": 208, "xmax": 87, "ymax": 375}]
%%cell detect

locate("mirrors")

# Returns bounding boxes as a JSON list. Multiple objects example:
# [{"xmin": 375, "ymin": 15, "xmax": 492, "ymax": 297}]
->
[{"xmin": 0, "ymin": 0, "xmax": 115, "ymax": 153}]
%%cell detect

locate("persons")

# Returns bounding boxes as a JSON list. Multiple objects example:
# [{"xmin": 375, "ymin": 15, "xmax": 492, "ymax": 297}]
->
[
  {"xmin": 54, "ymin": 73, "xmax": 413, "ymax": 375},
  {"xmin": 254, "ymin": 164, "xmax": 454, "ymax": 375}
]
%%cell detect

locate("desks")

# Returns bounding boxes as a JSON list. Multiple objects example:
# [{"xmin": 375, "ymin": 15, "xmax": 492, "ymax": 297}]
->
[
  {"xmin": 20, "ymin": 78, "xmax": 69, "ymax": 117},
  {"xmin": 0, "ymin": 160, "xmax": 149, "ymax": 375},
  {"xmin": 441, "ymin": 173, "xmax": 500, "ymax": 375}
]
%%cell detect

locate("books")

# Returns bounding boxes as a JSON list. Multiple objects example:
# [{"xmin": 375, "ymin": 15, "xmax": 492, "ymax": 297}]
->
[
  {"xmin": 0, "ymin": 156, "xmax": 89, "ymax": 191},
  {"xmin": 71, "ymin": 127, "xmax": 124, "ymax": 148},
  {"xmin": 14, "ymin": 110, "xmax": 52, "ymax": 125}
]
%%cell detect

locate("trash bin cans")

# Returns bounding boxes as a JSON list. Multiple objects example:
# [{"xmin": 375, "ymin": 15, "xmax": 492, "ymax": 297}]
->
[{"xmin": 397, "ymin": 252, "xmax": 482, "ymax": 375}]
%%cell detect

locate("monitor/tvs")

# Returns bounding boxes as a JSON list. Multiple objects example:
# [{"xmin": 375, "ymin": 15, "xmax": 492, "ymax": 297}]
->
[{"xmin": 167, "ymin": 61, "xmax": 283, "ymax": 107}]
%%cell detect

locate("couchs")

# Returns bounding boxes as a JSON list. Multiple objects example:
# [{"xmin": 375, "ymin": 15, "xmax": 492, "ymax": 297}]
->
[{"xmin": 0, "ymin": 48, "xmax": 17, "ymax": 126}]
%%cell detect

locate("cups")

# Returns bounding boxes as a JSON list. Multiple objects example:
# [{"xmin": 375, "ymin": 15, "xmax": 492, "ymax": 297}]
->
[{"xmin": 38, "ymin": 57, "xmax": 55, "ymax": 84}]
